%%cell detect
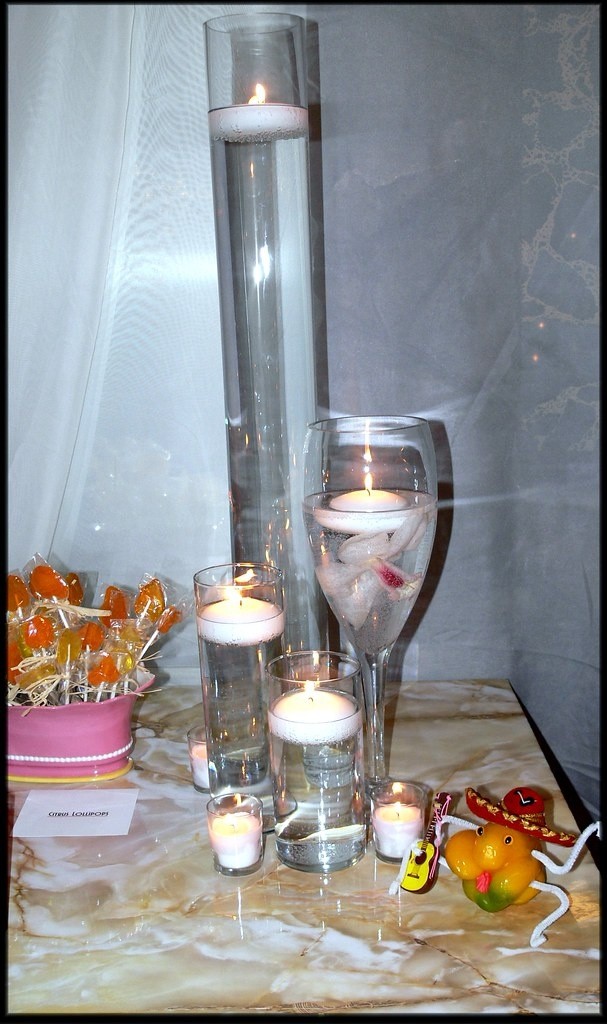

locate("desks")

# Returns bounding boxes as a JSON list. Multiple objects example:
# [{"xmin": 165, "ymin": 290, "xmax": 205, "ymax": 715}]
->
[{"xmin": 7, "ymin": 679, "xmax": 600, "ymax": 1015}]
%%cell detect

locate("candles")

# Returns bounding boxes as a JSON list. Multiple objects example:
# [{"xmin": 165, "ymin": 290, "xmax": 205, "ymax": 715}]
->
[
  {"xmin": 208, "ymin": 84, "xmax": 308, "ymax": 141},
  {"xmin": 191, "ymin": 744, "xmax": 210, "ymax": 788},
  {"xmin": 315, "ymin": 473, "xmax": 412, "ymax": 533},
  {"xmin": 372, "ymin": 783, "xmax": 425, "ymax": 857},
  {"xmin": 210, "ymin": 812, "xmax": 261, "ymax": 869},
  {"xmin": 270, "ymin": 680, "xmax": 362, "ymax": 743},
  {"xmin": 198, "ymin": 587, "xmax": 283, "ymax": 648}
]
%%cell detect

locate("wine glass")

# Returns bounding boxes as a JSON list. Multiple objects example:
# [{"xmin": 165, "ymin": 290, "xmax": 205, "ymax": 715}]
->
[{"xmin": 302, "ymin": 414, "xmax": 454, "ymax": 781}]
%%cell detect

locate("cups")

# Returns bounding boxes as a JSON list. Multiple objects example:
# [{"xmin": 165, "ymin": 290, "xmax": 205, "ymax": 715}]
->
[
  {"xmin": 206, "ymin": 793, "xmax": 264, "ymax": 876},
  {"xmin": 192, "ymin": 563, "xmax": 286, "ymax": 833},
  {"xmin": 265, "ymin": 651, "xmax": 361, "ymax": 873},
  {"xmin": 187, "ymin": 724, "xmax": 210, "ymax": 793},
  {"xmin": 370, "ymin": 782, "xmax": 424, "ymax": 865}
]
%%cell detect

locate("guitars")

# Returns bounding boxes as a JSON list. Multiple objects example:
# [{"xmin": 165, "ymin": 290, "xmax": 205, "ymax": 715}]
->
[{"xmin": 399, "ymin": 792, "xmax": 450, "ymax": 894}]
